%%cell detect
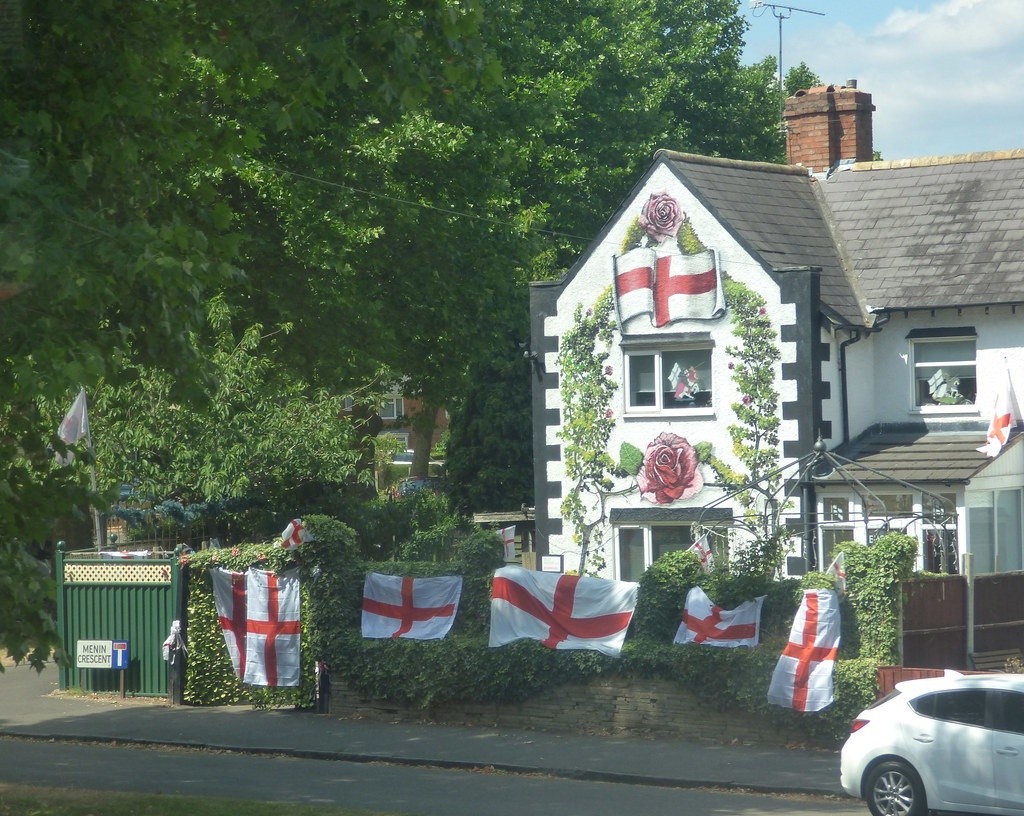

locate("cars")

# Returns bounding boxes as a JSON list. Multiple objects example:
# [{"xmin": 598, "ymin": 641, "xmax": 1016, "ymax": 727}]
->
[
  {"xmin": 379, "ymin": 447, "xmax": 445, "ymax": 467},
  {"xmin": 839, "ymin": 669, "xmax": 1024, "ymax": 816}
]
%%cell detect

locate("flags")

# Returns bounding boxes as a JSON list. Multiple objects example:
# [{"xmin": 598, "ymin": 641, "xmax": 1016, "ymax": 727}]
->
[
  {"xmin": 55, "ymin": 386, "xmax": 88, "ymax": 467},
  {"xmin": 361, "ymin": 571, "xmax": 463, "ymax": 640},
  {"xmin": 488, "ymin": 564, "xmax": 640, "ymax": 658},
  {"xmin": 974, "ymin": 362, "xmax": 1022, "ymax": 459},
  {"xmin": 767, "ymin": 589, "xmax": 841, "ymax": 715},
  {"xmin": 209, "ymin": 563, "xmax": 246, "ymax": 683},
  {"xmin": 686, "ymin": 535, "xmax": 715, "ymax": 577},
  {"xmin": 241, "ymin": 567, "xmax": 301, "ymax": 687},
  {"xmin": 671, "ymin": 585, "xmax": 767, "ymax": 649},
  {"xmin": 495, "ymin": 526, "xmax": 516, "ymax": 563},
  {"xmin": 279, "ymin": 517, "xmax": 307, "ymax": 549},
  {"xmin": 161, "ymin": 633, "xmax": 190, "ymax": 665},
  {"xmin": 826, "ymin": 549, "xmax": 846, "ymax": 603}
]
